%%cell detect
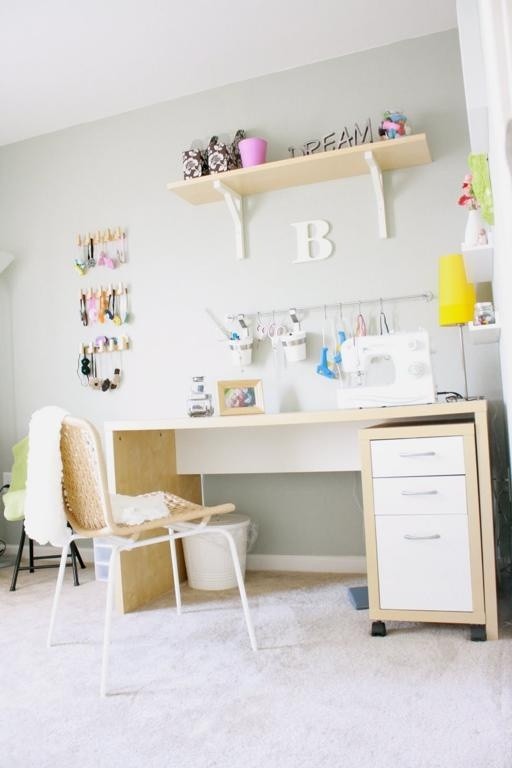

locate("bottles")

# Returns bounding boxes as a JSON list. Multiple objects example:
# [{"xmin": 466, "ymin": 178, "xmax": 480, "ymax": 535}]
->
[
  {"xmin": 474, "ymin": 301, "xmax": 496, "ymax": 326},
  {"xmin": 187, "ymin": 375, "xmax": 214, "ymax": 417}
]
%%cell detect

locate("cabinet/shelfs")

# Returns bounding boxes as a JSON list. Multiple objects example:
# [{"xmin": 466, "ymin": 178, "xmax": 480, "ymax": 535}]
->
[
  {"xmin": 102, "ymin": 398, "xmax": 499, "ymax": 641},
  {"xmin": 358, "ymin": 421, "xmax": 486, "ymax": 640},
  {"xmin": 463, "ymin": 245, "xmax": 500, "ymax": 343}
]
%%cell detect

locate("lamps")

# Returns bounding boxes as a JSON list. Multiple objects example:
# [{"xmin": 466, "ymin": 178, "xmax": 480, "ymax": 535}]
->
[{"xmin": 438, "ymin": 255, "xmax": 476, "ymax": 398}]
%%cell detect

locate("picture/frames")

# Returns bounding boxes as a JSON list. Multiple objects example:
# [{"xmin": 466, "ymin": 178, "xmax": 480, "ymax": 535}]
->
[{"xmin": 217, "ymin": 379, "xmax": 264, "ymax": 415}]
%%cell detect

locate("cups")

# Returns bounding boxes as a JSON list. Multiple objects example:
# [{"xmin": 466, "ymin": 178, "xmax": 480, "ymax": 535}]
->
[{"xmin": 238, "ymin": 138, "xmax": 267, "ymax": 167}]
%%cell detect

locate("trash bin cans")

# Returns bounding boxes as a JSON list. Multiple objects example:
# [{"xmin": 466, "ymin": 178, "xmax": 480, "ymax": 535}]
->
[{"xmin": 180, "ymin": 514, "xmax": 251, "ymax": 591}]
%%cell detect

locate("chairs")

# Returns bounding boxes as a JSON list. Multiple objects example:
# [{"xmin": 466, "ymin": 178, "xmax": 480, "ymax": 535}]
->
[
  {"xmin": 44, "ymin": 417, "xmax": 260, "ymax": 699},
  {"xmin": 3, "ymin": 437, "xmax": 85, "ymax": 590}
]
